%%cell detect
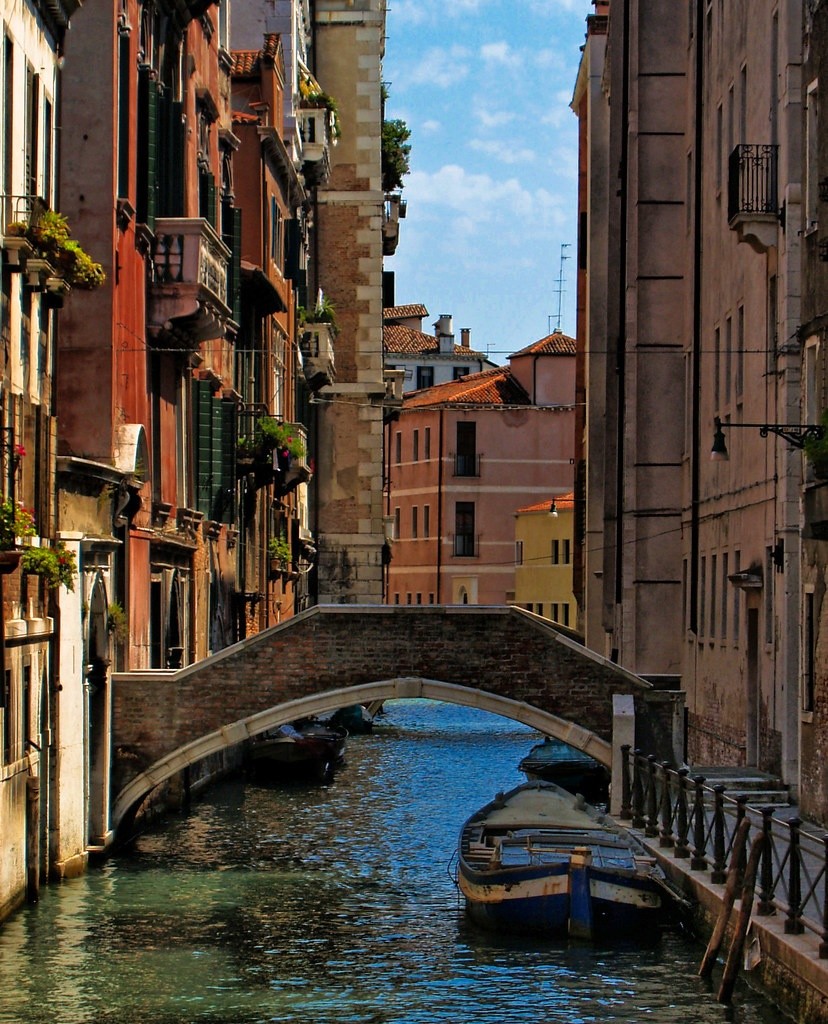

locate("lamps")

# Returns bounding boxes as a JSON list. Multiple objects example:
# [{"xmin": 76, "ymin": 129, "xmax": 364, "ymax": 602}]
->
[
  {"xmin": 709, "ymin": 416, "xmax": 828, "ymax": 462},
  {"xmin": 547, "ymin": 497, "xmax": 586, "ymax": 517}
]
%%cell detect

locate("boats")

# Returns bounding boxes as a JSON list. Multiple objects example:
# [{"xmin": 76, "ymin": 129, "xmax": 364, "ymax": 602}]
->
[
  {"xmin": 243, "ymin": 714, "xmax": 350, "ymax": 772},
  {"xmin": 448, "ymin": 779, "xmax": 672, "ymax": 925},
  {"xmin": 520, "ymin": 734, "xmax": 612, "ymax": 793}
]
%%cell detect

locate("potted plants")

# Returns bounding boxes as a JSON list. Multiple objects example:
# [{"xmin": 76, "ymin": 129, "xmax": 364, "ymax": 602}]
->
[
  {"xmin": 269, "ymin": 528, "xmax": 290, "ymax": 571},
  {"xmin": 8, "ymin": 208, "xmax": 104, "ymax": 289},
  {"xmin": 313, "ymin": 296, "xmax": 338, "ymax": 322},
  {"xmin": 300, "ymin": 90, "xmax": 330, "ymax": 108},
  {"xmin": 21, "ymin": 544, "xmax": 59, "ymax": 584}
]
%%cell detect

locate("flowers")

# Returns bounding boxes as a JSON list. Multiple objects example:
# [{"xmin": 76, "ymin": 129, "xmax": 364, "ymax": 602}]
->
[
  {"xmin": 12, "ymin": 444, "xmax": 26, "ymax": 457},
  {"xmin": 0, "ymin": 494, "xmax": 37, "ymax": 549},
  {"xmin": 282, "ymin": 423, "xmax": 304, "ymax": 460},
  {"xmin": 49, "ymin": 542, "xmax": 79, "ymax": 594}
]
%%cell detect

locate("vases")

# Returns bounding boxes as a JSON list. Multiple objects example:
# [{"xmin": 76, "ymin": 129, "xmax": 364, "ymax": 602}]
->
[{"xmin": 0, "ymin": 548, "xmax": 25, "ymax": 575}]
